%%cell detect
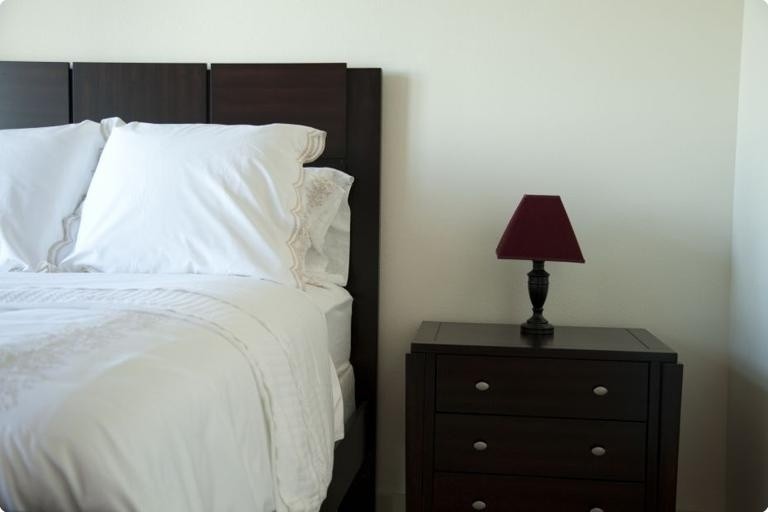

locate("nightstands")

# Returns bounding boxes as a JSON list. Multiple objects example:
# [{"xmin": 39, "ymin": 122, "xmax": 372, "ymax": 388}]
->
[{"xmin": 404, "ymin": 321, "xmax": 684, "ymax": 512}]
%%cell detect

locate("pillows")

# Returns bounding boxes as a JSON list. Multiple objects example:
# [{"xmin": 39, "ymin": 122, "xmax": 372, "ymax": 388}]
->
[
  {"xmin": 297, "ymin": 164, "xmax": 354, "ymax": 291},
  {"xmin": 50, "ymin": 118, "xmax": 328, "ymax": 291},
  {"xmin": 2, "ymin": 117, "xmax": 109, "ymax": 272}
]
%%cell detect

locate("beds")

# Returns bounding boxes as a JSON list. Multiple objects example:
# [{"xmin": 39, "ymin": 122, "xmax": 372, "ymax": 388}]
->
[{"xmin": 0, "ymin": 58, "xmax": 381, "ymax": 512}]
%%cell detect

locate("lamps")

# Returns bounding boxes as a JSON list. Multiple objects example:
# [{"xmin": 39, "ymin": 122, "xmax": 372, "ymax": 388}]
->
[{"xmin": 496, "ymin": 194, "xmax": 586, "ymax": 338}]
